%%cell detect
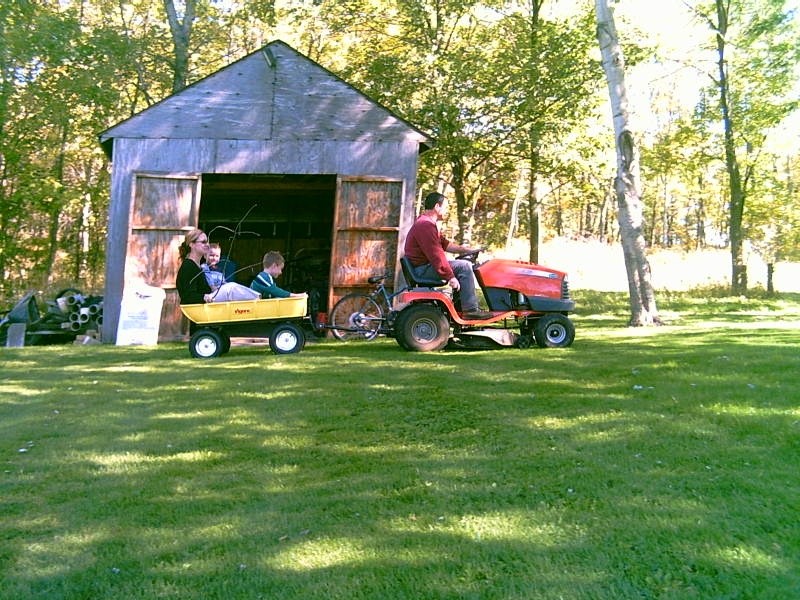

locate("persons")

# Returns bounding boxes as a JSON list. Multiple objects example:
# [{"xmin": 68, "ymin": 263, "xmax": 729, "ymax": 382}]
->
[
  {"xmin": 176, "ymin": 229, "xmax": 262, "ymax": 305},
  {"xmin": 402, "ymin": 191, "xmax": 494, "ymax": 321},
  {"xmin": 250, "ymin": 250, "xmax": 309, "ymax": 298}
]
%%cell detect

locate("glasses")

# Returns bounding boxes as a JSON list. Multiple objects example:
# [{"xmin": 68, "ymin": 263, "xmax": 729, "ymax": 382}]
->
[{"xmin": 193, "ymin": 240, "xmax": 207, "ymax": 244}]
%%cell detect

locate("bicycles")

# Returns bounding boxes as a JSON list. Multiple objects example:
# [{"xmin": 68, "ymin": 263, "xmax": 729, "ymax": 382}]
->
[
  {"xmin": 330, "ymin": 275, "xmax": 409, "ymax": 342},
  {"xmin": 280, "ymin": 255, "xmax": 328, "ymax": 337}
]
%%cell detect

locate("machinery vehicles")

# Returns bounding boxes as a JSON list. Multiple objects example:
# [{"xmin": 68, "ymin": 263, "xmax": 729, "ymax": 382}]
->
[{"xmin": 390, "ymin": 245, "xmax": 575, "ymax": 352}]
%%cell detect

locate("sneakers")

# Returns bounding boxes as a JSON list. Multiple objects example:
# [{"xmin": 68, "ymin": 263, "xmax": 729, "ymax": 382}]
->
[{"xmin": 462, "ymin": 308, "xmax": 493, "ymax": 319}]
